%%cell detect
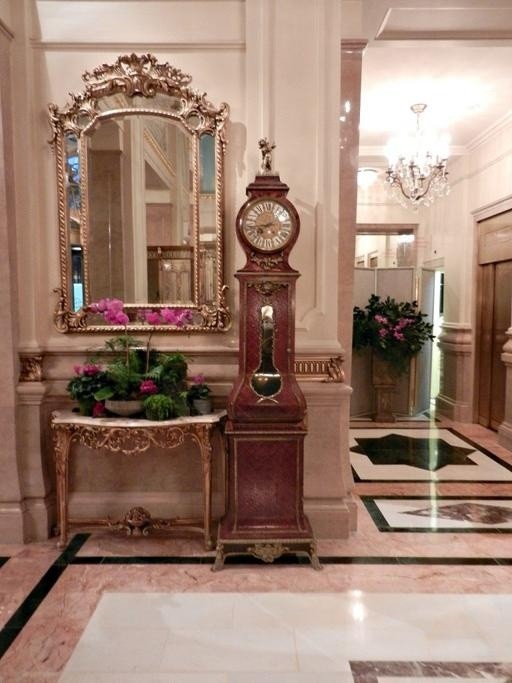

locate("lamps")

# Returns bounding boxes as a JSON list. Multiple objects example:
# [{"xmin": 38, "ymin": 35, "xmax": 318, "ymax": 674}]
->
[{"xmin": 380, "ymin": 101, "xmax": 453, "ymax": 211}]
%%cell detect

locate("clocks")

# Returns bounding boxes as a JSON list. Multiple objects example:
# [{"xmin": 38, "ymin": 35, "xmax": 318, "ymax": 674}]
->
[{"xmin": 210, "ymin": 134, "xmax": 324, "ymax": 575}]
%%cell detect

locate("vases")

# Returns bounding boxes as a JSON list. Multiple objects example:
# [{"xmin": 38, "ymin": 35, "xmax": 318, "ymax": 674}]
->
[{"xmin": 370, "ymin": 358, "xmax": 397, "ymax": 424}]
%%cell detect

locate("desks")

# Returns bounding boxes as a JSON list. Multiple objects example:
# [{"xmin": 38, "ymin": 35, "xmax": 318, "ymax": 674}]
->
[{"xmin": 49, "ymin": 408, "xmax": 228, "ymax": 552}]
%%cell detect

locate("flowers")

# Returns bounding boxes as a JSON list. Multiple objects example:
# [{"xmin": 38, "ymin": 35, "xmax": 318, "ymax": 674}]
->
[
  {"xmin": 66, "ymin": 298, "xmax": 214, "ymax": 418},
  {"xmin": 351, "ymin": 293, "xmax": 437, "ymax": 380}
]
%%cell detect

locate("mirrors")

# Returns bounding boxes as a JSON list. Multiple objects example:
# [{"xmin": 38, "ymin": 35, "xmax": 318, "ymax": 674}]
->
[{"xmin": 45, "ymin": 51, "xmax": 235, "ymax": 338}]
